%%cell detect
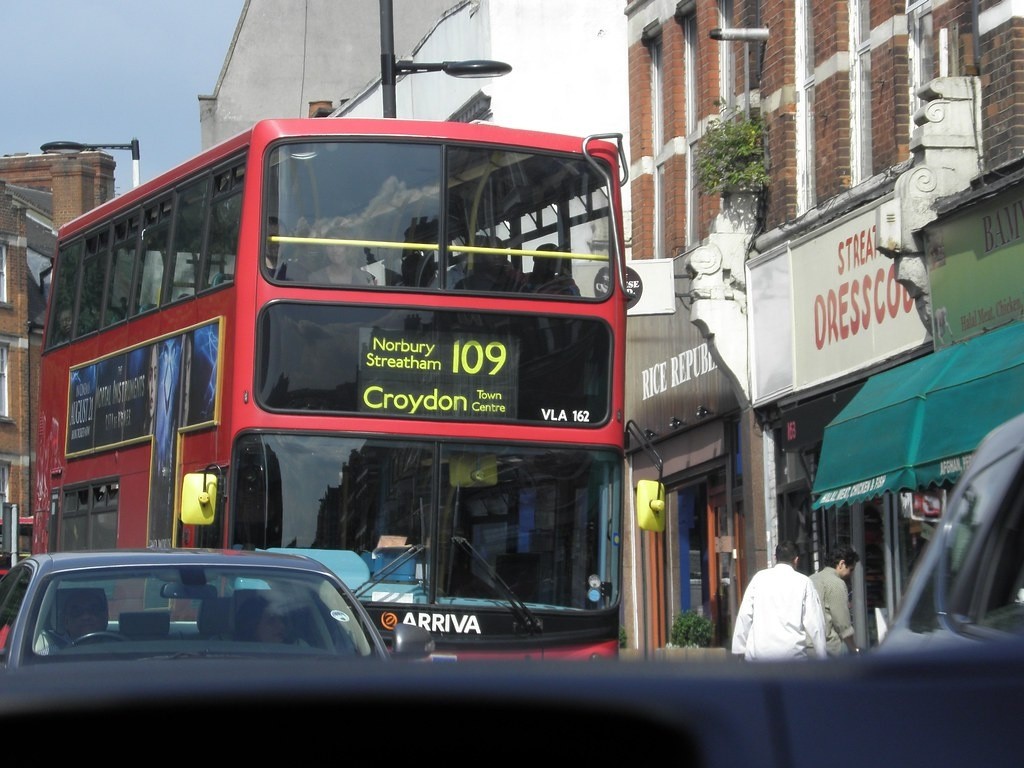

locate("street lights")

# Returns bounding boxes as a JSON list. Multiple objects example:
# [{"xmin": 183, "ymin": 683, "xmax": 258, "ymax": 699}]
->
[
  {"xmin": 378, "ymin": 0, "xmax": 512, "ymax": 121},
  {"xmin": 38, "ymin": 140, "xmax": 142, "ymax": 190}
]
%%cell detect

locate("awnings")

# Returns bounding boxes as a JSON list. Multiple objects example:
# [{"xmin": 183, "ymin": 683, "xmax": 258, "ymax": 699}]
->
[{"xmin": 812, "ymin": 322, "xmax": 1024, "ymax": 508}]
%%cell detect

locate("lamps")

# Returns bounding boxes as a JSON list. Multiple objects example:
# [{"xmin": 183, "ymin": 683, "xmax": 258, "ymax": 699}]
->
[
  {"xmin": 644, "ymin": 429, "xmax": 657, "ymax": 440},
  {"xmin": 668, "ymin": 416, "xmax": 687, "ymax": 430},
  {"xmin": 696, "ymin": 406, "xmax": 715, "ymax": 419}
]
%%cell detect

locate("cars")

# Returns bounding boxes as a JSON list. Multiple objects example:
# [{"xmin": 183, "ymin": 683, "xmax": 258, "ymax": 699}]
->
[{"xmin": 1, "ymin": 551, "xmax": 435, "ymax": 675}]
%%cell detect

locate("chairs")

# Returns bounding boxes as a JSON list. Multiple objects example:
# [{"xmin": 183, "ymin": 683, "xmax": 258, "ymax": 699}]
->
[
  {"xmin": 34, "ymin": 588, "xmax": 124, "ymax": 651},
  {"xmin": 187, "ymin": 595, "xmax": 294, "ymax": 642}
]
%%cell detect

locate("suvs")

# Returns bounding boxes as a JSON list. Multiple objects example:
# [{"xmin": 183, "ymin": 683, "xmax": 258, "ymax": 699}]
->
[{"xmin": 860, "ymin": 412, "xmax": 1024, "ymax": 646}]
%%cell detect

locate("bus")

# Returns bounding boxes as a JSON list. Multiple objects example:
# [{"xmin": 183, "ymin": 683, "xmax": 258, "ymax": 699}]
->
[{"xmin": 34, "ymin": 116, "xmax": 667, "ymax": 665}]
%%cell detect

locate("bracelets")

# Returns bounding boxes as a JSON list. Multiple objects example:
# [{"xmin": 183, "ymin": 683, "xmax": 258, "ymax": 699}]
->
[{"xmin": 850, "ymin": 648, "xmax": 860, "ymax": 653}]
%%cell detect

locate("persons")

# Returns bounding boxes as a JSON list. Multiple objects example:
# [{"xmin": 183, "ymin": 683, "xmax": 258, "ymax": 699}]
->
[
  {"xmin": 732, "ymin": 541, "xmax": 864, "ymax": 662},
  {"xmin": 265, "ymin": 216, "xmax": 580, "ymax": 296},
  {"xmin": 237, "ymin": 598, "xmax": 344, "ymax": 664},
  {"xmin": 51, "ymin": 591, "xmax": 123, "ymax": 662},
  {"xmin": 360, "ymin": 523, "xmax": 416, "ymax": 582},
  {"xmin": 138, "ymin": 332, "xmax": 195, "ymax": 537},
  {"xmin": 56, "ymin": 308, "xmax": 71, "ymax": 346}
]
